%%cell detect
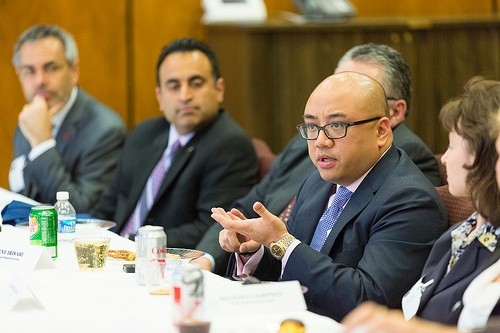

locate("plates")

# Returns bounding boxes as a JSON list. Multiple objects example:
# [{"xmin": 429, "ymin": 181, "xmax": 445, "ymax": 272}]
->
[
  {"xmin": 166, "ymin": 245, "xmax": 206, "ymax": 265},
  {"xmin": 16, "ymin": 218, "xmax": 118, "ymax": 237}
]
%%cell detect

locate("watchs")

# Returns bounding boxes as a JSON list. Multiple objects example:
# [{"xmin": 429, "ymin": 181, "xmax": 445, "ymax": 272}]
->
[{"xmin": 270, "ymin": 234, "xmax": 297, "ymax": 260}]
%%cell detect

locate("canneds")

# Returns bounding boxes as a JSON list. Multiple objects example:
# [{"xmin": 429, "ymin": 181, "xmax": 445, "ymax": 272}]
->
[
  {"xmin": 29, "ymin": 205, "xmax": 59, "ymax": 260},
  {"xmin": 134, "ymin": 225, "xmax": 167, "ymax": 287},
  {"xmin": 169, "ymin": 264, "xmax": 204, "ymax": 328}
]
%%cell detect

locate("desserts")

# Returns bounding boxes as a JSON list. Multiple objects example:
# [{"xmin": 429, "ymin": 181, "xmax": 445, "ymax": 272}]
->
[{"xmin": 279, "ymin": 320, "xmax": 304, "ymax": 332}]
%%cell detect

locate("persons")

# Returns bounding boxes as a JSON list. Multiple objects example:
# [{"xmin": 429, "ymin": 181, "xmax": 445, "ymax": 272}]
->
[
  {"xmin": 12, "ymin": 26, "xmax": 127, "ymax": 215},
  {"xmin": 88, "ymin": 36, "xmax": 261, "ymax": 279},
  {"xmin": 210, "ymin": 43, "xmax": 500, "ymax": 333}
]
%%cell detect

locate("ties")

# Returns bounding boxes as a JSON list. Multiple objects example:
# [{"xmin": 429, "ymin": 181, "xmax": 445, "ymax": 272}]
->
[
  {"xmin": 119, "ymin": 140, "xmax": 181, "ymax": 240},
  {"xmin": 309, "ymin": 186, "xmax": 353, "ymax": 253}
]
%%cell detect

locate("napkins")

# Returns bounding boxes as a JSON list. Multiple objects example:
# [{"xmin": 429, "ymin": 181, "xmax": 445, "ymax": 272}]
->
[{"xmin": 1, "ymin": 200, "xmax": 34, "ymax": 227}]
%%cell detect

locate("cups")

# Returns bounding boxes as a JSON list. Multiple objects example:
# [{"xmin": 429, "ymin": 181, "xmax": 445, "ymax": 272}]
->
[{"xmin": 71, "ymin": 235, "xmax": 111, "ymax": 270}]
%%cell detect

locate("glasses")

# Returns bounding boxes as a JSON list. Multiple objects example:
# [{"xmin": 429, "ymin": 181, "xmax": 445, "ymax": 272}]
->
[{"xmin": 296, "ymin": 116, "xmax": 381, "ymax": 141}]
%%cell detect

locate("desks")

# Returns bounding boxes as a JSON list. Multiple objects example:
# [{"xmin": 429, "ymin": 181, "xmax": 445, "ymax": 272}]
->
[{"xmin": 0, "ymin": 185, "xmax": 340, "ymax": 333}]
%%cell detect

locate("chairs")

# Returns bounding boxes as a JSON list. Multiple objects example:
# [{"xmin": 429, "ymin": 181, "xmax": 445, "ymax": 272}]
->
[{"xmin": 427, "ymin": 154, "xmax": 476, "ymax": 228}]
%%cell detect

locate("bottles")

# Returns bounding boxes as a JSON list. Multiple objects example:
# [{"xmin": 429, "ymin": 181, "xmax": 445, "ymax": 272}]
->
[{"xmin": 51, "ymin": 191, "xmax": 77, "ymax": 258}]
